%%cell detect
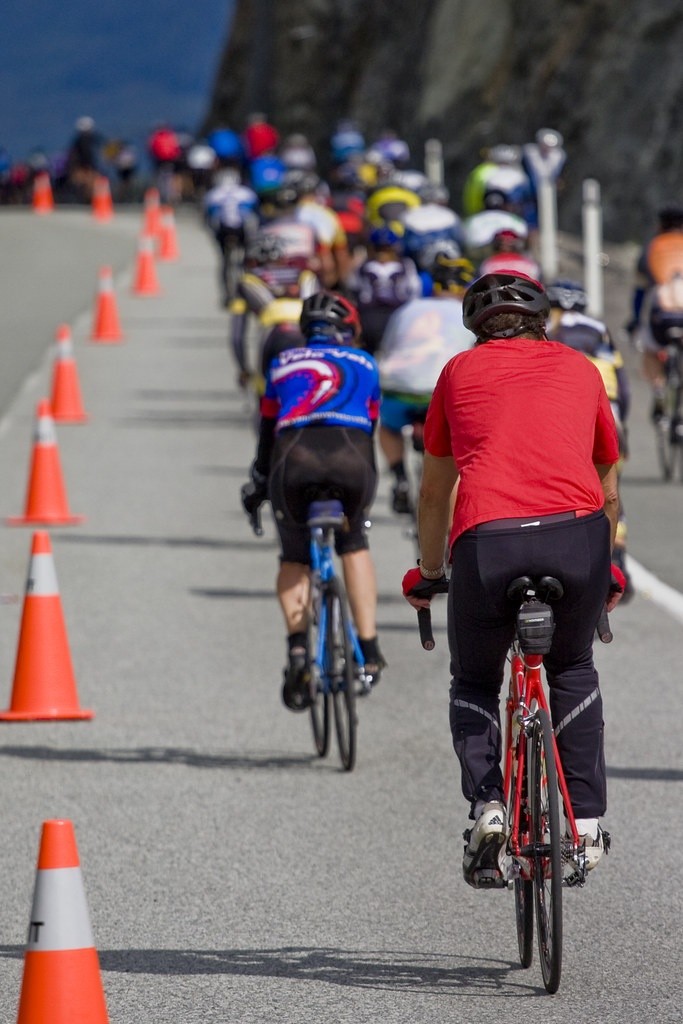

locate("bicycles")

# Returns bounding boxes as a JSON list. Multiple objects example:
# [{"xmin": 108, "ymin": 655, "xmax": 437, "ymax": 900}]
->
[
  {"xmin": 239, "ymin": 479, "xmax": 363, "ymax": 772},
  {"xmin": 411, "ymin": 576, "xmax": 612, "ymax": 998},
  {"xmin": 650, "ymin": 326, "xmax": 682, "ymax": 480}
]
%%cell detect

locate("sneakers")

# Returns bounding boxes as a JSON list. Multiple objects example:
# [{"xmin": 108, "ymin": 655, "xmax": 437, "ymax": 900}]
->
[
  {"xmin": 462, "ymin": 803, "xmax": 507, "ymax": 888},
  {"xmin": 564, "ymin": 823, "xmax": 605, "ymax": 871}
]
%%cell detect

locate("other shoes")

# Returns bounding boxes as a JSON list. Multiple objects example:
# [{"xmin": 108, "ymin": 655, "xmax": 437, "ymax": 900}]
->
[
  {"xmin": 392, "ymin": 479, "xmax": 415, "ymax": 513},
  {"xmin": 355, "ymin": 657, "xmax": 385, "ymax": 696},
  {"xmin": 283, "ymin": 645, "xmax": 310, "ymax": 710}
]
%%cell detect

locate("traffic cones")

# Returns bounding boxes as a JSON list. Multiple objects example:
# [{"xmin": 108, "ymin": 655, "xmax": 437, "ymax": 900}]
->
[
  {"xmin": 143, "ymin": 186, "xmax": 162, "ymax": 238},
  {"xmin": 17, "ymin": 818, "xmax": 111, "ymax": 1023},
  {"xmin": 133, "ymin": 236, "xmax": 160, "ymax": 296},
  {"xmin": 45, "ymin": 323, "xmax": 89, "ymax": 421},
  {"xmin": 93, "ymin": 177, "xmax": 114, "ymax": 222},
  {"xmin": 6, "ymin": 397, "xmax": 89, "ymax": 527},
  {"xmin": 159, "ymin": 205, "xmax": 179, "ymax": 261},
  {"xmin": 33, "ymin": 171, "xmax": 55, "ymax": 217},
  {"xmin": 0, "ymin": 529, "xmax": 96, "ymax": 722},
  {"xmin": 92, "ymin": 267, "xmax": 124, "ymax": 343}
]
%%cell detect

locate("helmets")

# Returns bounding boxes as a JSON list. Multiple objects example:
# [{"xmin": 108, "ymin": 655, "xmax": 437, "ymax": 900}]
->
[
  {"xmin": 433, "ymin": 251, "xmax": 476, "ymax": 295},
  {"xmin": 271, "ymin": 184, "xmax": 301, "ymax": 208},
  {"xmin": 489, "ymin": 145, "xmax": 520, "ymax": 162},
  {"xmin": 482, "ymin": 189, "xmax": 513, "ymax": 209},
  {"xmin": 462, "ymin": 269, "xmax": 551, "ymax": 331},
  {"xmin": 480, "ymin": 251, "xmax": 541, "ymax": 282},
  {"xmin": 495, "ymin": 230, "xmax": 522, "ymax": 248},
  {"xmin": 300, "ymin": 290, "xmax": 363, "ymax": 339},
  {"xmin": 418, "ymin": 182, "xmax": 448, "ymax": 203},
  {"xmin": 546, "ymin": 280, "xmax": 588, "ymax": 309},
  {"xmin": 370, "ymin": 227, "xmax": 397, "ymax": 248}
]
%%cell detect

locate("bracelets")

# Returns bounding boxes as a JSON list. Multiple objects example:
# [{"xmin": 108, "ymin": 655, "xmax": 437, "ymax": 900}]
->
[{"xmin": 420, "ymin": 558, "xmax": 445, "ymax": 576}]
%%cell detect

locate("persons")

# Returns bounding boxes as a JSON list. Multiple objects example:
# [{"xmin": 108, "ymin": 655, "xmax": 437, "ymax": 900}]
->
[
  {"xmin": 0, "ymin": 110, "xmax": 636, "ymax": 607},
  {"xmin": 239, "ymin": 288, "xmax": 387, "ymax": 712},
  {"xmin": 624, "ymin": 205, "xmax": 683, "ymax": 448},
  {"xmin": 400, "ymin": 269, "xmax": 629, "ymax": 889}
]
